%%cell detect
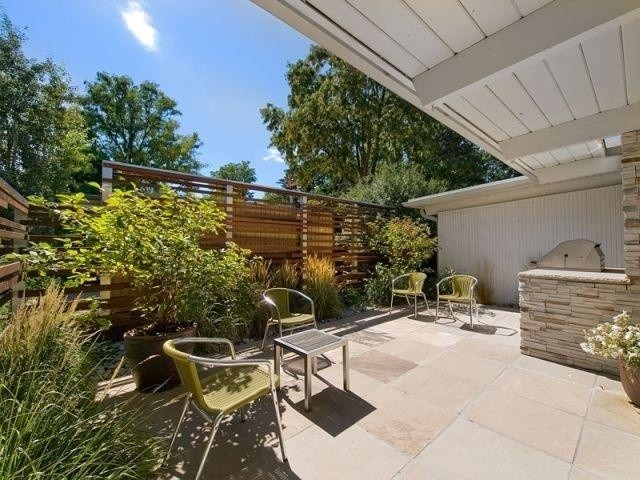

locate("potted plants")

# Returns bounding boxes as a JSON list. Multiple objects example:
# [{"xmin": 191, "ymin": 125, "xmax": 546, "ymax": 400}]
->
[{"xmin": 17, "ymin": 180, "xmax": 262, "ymax": 395}]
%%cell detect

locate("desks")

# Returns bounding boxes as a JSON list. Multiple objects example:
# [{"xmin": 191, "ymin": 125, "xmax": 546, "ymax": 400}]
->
[{"xmin": 273, "ymin": 328, "xmax": 350, "ymax": 413}]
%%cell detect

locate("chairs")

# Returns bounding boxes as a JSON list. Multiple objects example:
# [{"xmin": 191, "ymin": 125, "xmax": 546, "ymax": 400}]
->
[
  {"xmin": 388, "ymin": 271, "xmax": 431, "ymax": 320},
  {"xmin": 159, "ymin": 331, "xmax": 287, "ymax": 478},
  {"xmin": 434, "ymin": 274, "xmax": 480, "ymax": 330},
  {"xmin": 262, "ymin": 288, "xmax": 318, "ymax": 351}
]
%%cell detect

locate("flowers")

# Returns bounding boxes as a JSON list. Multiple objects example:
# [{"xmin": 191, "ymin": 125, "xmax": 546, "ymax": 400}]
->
[{"xmin": 579, "ymin": 310, "xmax": 640, "ymax": 360}]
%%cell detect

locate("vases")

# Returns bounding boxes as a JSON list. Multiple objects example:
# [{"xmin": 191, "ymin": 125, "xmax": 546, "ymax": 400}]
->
[{"xmin": 617, "ymin": 355, "xmax": 640, "ymax": 407}]
